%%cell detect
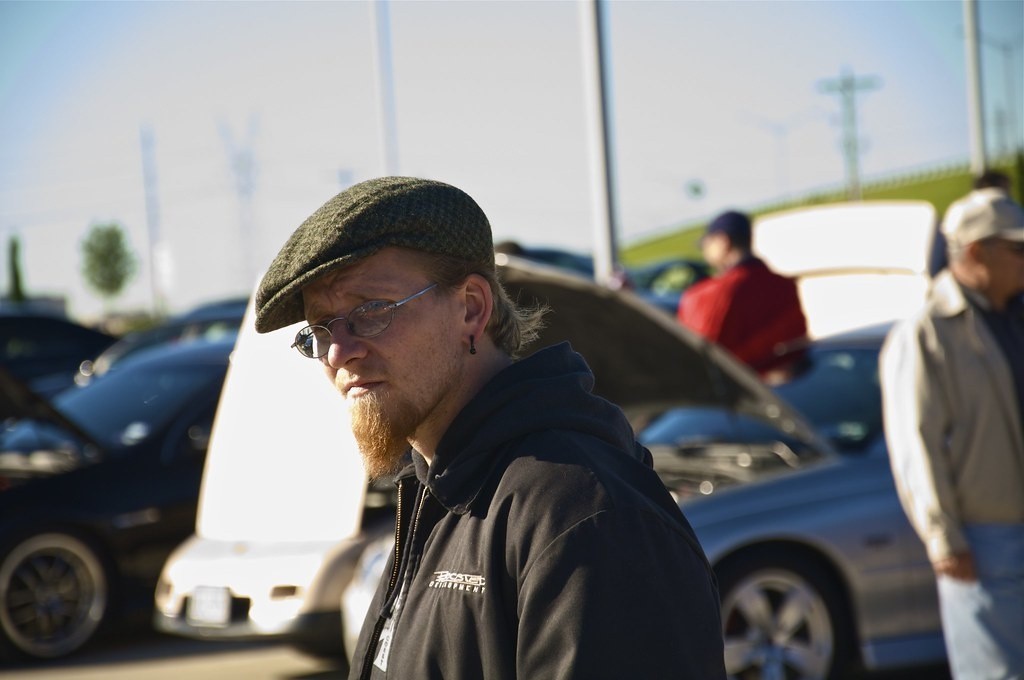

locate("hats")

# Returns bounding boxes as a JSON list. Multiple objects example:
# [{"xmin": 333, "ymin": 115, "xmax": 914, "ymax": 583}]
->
[
  {"xmin": 254, "ymin": 177, "xmax": 494, "ymax": 335},
  {"xmin": 938, "ymin": 187, "xmax": 1023, "ymax": 244}
]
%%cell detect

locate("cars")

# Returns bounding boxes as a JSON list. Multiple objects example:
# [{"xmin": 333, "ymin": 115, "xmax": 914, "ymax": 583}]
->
[{"xmin": 0, "ymin": 249, "xmax": 952, "ymax": 680}]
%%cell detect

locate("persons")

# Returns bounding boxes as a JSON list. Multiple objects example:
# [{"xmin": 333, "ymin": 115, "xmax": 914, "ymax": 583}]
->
[
  {"xmin": 976, "ymin": 172, "xmax": 1009, "ymax": 191},
  {"xmin": 254, "ymin": 177, "xmax": 730, "ymax": 680},
  {"xmin": 678, "ymin": 212, "xmax": 809, "ymax": 367},
  {"xmin": 877, "ymin": 188, "xmax": 1024, "ymax": 679}
]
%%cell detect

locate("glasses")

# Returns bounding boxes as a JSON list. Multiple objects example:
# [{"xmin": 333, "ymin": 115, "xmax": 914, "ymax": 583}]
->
[{"xmin": 290, "ymin": 281, "xmax": 442, "ymax": 360}]
